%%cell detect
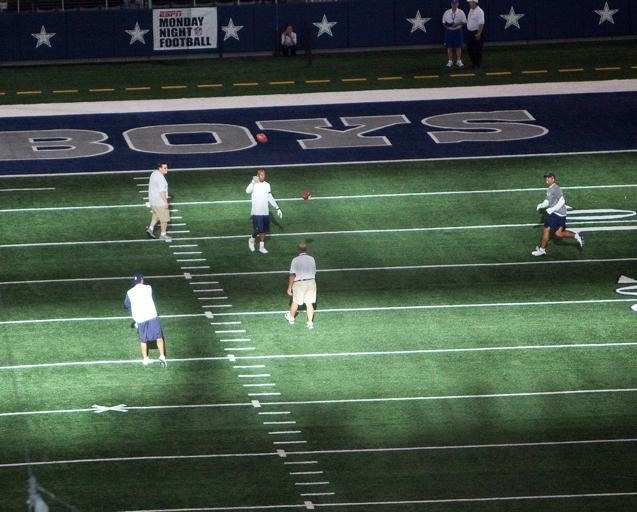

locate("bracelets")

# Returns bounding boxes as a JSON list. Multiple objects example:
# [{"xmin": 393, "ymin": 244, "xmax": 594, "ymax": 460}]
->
[{"xmin": 276, "ymin": 208, "xmax": 280, "ymax": 210}]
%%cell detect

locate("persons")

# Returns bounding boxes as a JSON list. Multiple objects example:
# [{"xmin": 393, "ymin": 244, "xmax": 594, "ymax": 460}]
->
[
  {"xmin": 532, "ymin": 171, "xmax": 584, "ymax": 256},
  {"xmin": 281, "ymin": 25, "xmax": 298, "ymax": 57},
  {"xmin": 284, "ymin": 240, "xmax": 317, "ymax": 330},
  {"xmin": 123, "ymin": 273, "xmax": 168, "ymax": 368},
  {"xmin": 442, "ymin": 1, "xmax": 467, "ymax": 68},
  {"xmin": 245, "ymin": 168, "xmax": 282, "ymax": 254},
  {"xmin": 146, "ymin": 161, "xmax": 172, "ymax": 240},
  {"xmin": 467, "ymin": 0, "xmax": 485, "ymax": 68}
]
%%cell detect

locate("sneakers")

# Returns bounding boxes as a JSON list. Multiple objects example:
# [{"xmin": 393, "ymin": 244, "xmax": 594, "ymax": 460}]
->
[
  {"xmin": 259, "ymin": 248, "xmax": 270, "ymax": 254},
  {"xmin": 157, "ymin": 355, "xmax": 167, "ymax": 368},
  {"xmin": 146, "ymin": 228, "xmax": 155, "ymax": 239},
  {"xmin": 159, "ymin": 234, "xmax": 172, "ymax": 241},
  {"xmin": 530, "ymin": 246, "xmax": 547, "ymax": 257},
  {"xmin": 248, "ymin": 238, "xmax": 255, "ymax": 252},
  {"xmin": 445, "ymin": 60, "xmax": 464, "ymax": 69},
  {"xmin": 284, "ymin": 310, "xmax": 295, "ymax": 324},
  {"xmin": 143, "ymin": 359, "xmax": 154, "ymax": 367},
  {"xmin": 305, "ymin": 323, "xmax": 314, "ymax": 330},
  {"xmin": 574, "ymin": 230, "xmax": 585, "ymax": 250}
]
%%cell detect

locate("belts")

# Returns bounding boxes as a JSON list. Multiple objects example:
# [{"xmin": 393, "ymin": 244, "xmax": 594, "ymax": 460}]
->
[{"xmin": 294, "ymin": 278, "xmax": 314, "ymax": 281}]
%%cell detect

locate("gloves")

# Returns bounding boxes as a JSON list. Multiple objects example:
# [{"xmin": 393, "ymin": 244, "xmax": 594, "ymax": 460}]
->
[
  {"xmin": 546, "ymin": 208, "xmax": 553, "ymax": 214},
  {"xmin": 251, "ymin": 176, "xmax": 259, "ymax": 184},
  {"xmin": 536, "ymin": 203, "xmax": 543, "ymax": 211},
  {"xmin": 276, "ymin": 210, "xmax": 282, "ymax": 219}
]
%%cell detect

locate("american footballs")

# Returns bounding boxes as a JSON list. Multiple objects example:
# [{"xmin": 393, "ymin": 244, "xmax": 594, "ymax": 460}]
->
[
  {"xmin": 303, "ymin": 193, "xmax": 309, "ymax": 198},
  {"xmin": 256, "ymin": 133, "xmax": 268, "ymax": 144}
]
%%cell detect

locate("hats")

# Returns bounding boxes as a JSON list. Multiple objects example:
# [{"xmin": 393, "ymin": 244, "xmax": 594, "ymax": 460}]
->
[
  {"xmin": 133, "ymin": 273, "xmax": 143, "ymax": 284},
  {"xmin": 543, "ymin": 172, "xmax": 555, "ymax": 177}
]
